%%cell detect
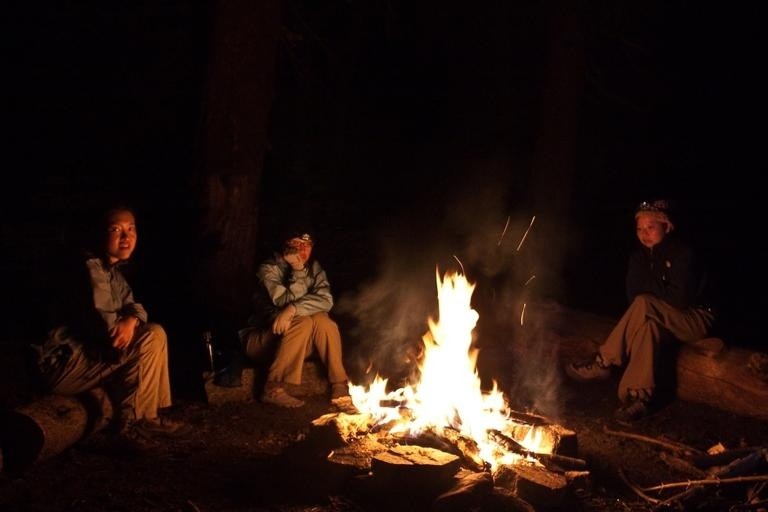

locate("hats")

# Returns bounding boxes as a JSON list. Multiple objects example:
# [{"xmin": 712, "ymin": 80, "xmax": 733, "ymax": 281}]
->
[{"xmin": 634, "ymin": 200, "xmax": 670, "ymax": 223}]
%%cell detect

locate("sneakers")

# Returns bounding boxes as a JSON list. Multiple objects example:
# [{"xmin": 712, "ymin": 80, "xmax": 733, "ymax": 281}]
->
[
  {"xmin": 569, "ymin": 351, "xmax": 612, "ymax": 380},
  {"xmin": 612, "ymin": 394, "xmax": 648, "ymax": 423},
  {"xmin": 110, "ymin": 423, "xmax": 168, "ymax": 456},
  {"xmin": 331, "ymin": 384, "xmax": 349, "ymax": 403},
  {"xmin": 139, "ymin": 413, "xmax": 195, "ymax": 441},
  {"xmin": 260, "ymin": 386, "xmax": 305, "ymax": 408}
]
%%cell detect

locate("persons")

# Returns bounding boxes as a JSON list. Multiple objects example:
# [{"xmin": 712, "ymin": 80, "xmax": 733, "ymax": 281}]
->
[
  {"xmin": 567, "ymin": 192, "xmax": 718, "ymax": 428},
  {"xmin": 235, "ymin": 222, "xmax": 361, "ymax": 412},
  {"xmin": 26, "ymin": 201, "xmax": 192, "ymax": 457}
]
